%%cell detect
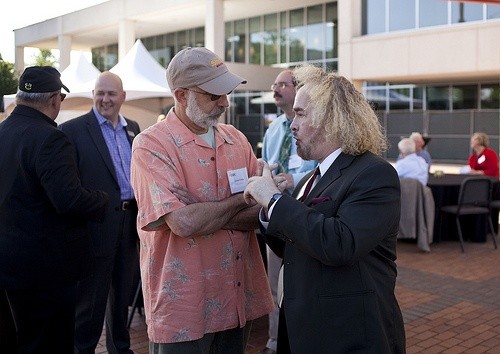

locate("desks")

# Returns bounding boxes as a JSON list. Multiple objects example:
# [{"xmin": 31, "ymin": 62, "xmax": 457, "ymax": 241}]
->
[{"xmin": 425, "ymin": 172, "xmax": 499, "ymax": 242}]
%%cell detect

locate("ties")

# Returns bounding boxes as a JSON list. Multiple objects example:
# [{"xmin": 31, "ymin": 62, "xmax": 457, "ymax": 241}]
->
[
  {"xmin": 297, "ymin": 167, "xmax": 321, "ymax": 203},
  {"xmin": 276, "ymin": 121, "xmax": 292, "ymax": 196}
]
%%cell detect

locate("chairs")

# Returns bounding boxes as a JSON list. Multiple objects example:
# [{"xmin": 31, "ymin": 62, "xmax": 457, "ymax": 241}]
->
[{"xmin": 397, "ymin": 177, "xmax": 500, "ymax": 251}]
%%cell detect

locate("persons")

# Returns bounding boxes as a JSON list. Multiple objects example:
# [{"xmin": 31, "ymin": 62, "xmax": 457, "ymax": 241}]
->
[
  {"xmin": 128, "ymin": 47, "xmax": 266, "ymax": 354},
  {"xmin": 259, "ymin": 69, "xmax": 317, "ymax": 354},
  {"xmin": 390, "ymin": 132, "xmax": 431, "ymax": 186},
  {"xmin": 466, "ymin": 133, "xmax": 500, "ymax": 179},
  {"xmin": 244, "ymin": 65, "xmax": 405, "ymax": 354},
  {"xmin": 59, "ymin": 71, "xmax": 141, "ymax": 354},
  {"xmin": 0, "ymin": 66, "xmax": 110, "ymax": 354}
]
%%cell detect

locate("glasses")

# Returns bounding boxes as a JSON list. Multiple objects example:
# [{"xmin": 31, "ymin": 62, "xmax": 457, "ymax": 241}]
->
[
  {"xmin": 271, "ymin": 82, "xmax": 295, "ymax": 91},
  {"xmin": 49, "ymin": 93, "xmax": 67, "ymax": 102},
  {"xmin": 183, "ymin": 88, "xmax": 233, "ymax": 101}
]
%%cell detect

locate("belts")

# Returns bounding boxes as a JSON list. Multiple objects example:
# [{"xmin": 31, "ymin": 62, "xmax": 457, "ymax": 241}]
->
[{"xmin": 119, "ymin": 200, "xmax": 137, "ymax": 211}]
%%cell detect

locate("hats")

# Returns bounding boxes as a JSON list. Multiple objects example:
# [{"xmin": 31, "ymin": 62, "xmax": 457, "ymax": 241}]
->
[
  {"xmin": 19, "ymin": 66, "xmax": 70, "ymax": 93},
  {"xmin": 166, "ymin": 47, "xmax": 247, "ymax": 96}
]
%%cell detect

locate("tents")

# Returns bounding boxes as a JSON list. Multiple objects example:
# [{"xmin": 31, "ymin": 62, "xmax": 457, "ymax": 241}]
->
[{"xmin": 4, "ymin": 41, "xmax": 231, "ymax": 133}]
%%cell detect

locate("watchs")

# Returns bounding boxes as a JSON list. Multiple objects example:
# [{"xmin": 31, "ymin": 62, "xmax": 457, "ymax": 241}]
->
[{"xmin": 267, "ymin": 193, "xmax": 282, "ymax": 211}]
